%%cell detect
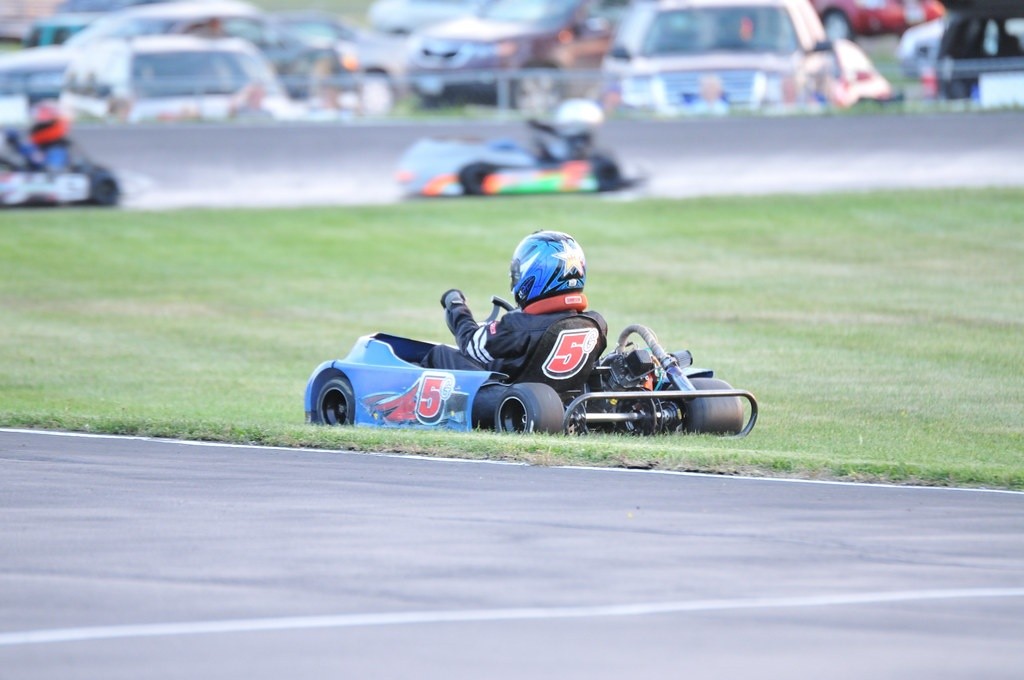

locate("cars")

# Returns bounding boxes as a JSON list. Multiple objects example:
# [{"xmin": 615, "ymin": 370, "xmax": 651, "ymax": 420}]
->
[
  {"xmin": 902, "ymin": 18, "xmax": 1023, "ymax": 99},
  {"xmin": 919, "ymin": 0, "xmax": 1024, "ymax": 107},
  {"xmin": 0, "ymin": 0, "xmax": 402, "ymax": 119},
  {"xmin": 404, "ymin": 0, "xmax": 631, "ymax": 116},
  {"xmin": 368, "ymin": 1, "xmax": 494, "ymax": 42},
  {"xmin": 597, "ymin": 0, "xmax": 835, "ymax": 114},
  {"xmin": 809, "ymin": 0, "xmax": 946, "ymax": 43}
]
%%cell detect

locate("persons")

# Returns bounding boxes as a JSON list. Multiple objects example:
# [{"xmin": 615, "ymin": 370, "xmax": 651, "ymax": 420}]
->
[
  {"xmin": 525, "ymin": 96, "xmax": 605, "ymax": 170},
  {"xmin": 96, "ymin": 75, "xmax": 364, "ymax": 123},
  {"xmin": 600, "ymin": 57, "xmax": 848, "ymax": 110},
  {"xmin": 419, "ymin": 229, "xmax": 607, "ymax": 385},
  {"xmin": 1, "ymin": 104, "xmax": 75, "ymax": 178}
]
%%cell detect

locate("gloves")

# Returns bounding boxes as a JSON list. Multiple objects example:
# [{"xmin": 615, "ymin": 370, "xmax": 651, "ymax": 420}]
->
[{"xmin": 440, "ymin": 289, "xmax": 466, "ymax": 310}]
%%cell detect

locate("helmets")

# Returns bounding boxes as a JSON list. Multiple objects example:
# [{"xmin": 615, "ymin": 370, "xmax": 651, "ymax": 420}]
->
[
  {"xmin": 510, "ymin": 230, "xmax": 587, "ymax": 308},
  {"xmin": 553, "ymin": 98, "xmax": 604, "ymax": 140},
  {"xmin": 32, "ymin": 106, "xmax": 69, "ymax": 147}
]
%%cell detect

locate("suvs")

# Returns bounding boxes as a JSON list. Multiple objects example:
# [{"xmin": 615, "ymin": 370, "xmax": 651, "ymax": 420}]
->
[{"xmin": 61, "ymin": 34, "xmax": 292, "ymax": 127}]
